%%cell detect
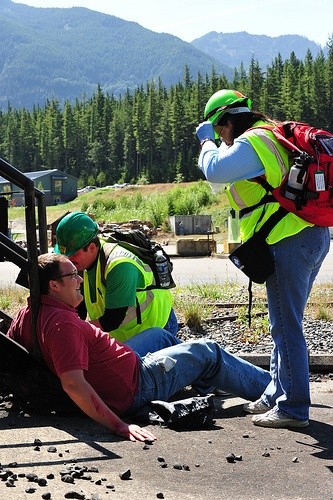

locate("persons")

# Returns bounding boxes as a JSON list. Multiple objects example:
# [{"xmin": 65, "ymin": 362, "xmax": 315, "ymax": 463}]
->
[
  {"xmin": 53, "ymin": 212, "xmax": 178, "ymax": 343},
  {"xmin": 196, "ymin": 90, "xmax": 331, "ymax": 426},
  {"xmin": 9, "ymin": 252, "xmax": 272, "ymax": 442}
]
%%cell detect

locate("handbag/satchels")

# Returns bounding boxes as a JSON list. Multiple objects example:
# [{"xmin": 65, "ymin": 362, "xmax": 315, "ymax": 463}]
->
[
  {"xmin": 133, "ymin": 387, "xmax": 217, "ymax": 431},
  {"xmin": 228, "ymin": 233, "xmax": 274, "ymax": 284}
]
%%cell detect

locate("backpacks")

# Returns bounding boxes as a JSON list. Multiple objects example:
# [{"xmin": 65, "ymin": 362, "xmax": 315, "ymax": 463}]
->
[
  {"xmin": 244, "ymin": 117, "xmax": 333, "ymax": 227},
  {"xmin": 98, "ymin": 230, "xmax": 176, "ymax": 292}
]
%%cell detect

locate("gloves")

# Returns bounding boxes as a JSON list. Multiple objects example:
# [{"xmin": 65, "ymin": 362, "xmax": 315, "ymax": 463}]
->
[{"xmin": 196, "ymin": 121, "xmax": 215, "ymax": 145}]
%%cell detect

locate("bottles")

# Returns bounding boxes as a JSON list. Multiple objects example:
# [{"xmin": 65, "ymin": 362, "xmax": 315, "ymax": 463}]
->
[
  {"xmin": 285, "ymin": 156, "xmax": 306, "ymax": 201},
  {"xmin": 156, "ymin": 250, "xmax": 170, "ymax": 287}
]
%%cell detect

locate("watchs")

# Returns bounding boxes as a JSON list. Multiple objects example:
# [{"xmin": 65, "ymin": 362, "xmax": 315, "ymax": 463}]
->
[{"xmin": 200, "ymin": 138, "xmax": 214, "ymax": 146}]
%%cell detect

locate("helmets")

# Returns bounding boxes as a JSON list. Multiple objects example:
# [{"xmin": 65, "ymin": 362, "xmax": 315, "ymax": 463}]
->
[
  {"xmin": 204, "ymin": 89, "xmax": 251, "ymax": 139},
  {"xmin": 54, "ymin": 212, "xmax": 99, "ymax": 257}
]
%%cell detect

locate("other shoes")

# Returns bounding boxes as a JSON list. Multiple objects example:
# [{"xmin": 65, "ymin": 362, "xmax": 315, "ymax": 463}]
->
[{"xmin": 217, "ymin": 388, "xmax": 238, "ymax": 397}]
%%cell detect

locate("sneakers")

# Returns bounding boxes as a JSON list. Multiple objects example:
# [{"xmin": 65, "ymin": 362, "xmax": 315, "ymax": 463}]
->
[
  {"xmin": 243, "ymin": 400, "xmax": 271, "ymax": 413},
  {"xmin": 251, "ymin": 405, "xmax": 309, "ymax": 427}
]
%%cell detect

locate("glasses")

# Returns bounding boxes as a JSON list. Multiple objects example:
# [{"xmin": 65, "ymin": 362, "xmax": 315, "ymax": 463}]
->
[{"xmin": 48, "ymin": 270, "xmax": 78, "ymax": 289}]
